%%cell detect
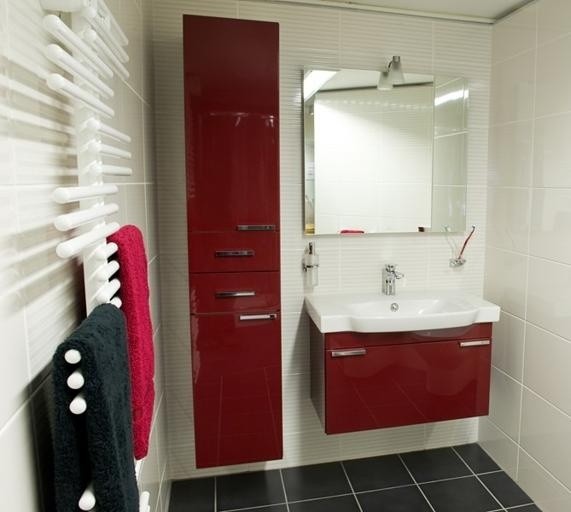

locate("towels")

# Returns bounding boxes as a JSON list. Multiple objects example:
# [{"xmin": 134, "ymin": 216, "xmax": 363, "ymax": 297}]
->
[
  {"xmin": 48, "ymin": 303, "xmax": 140, "ymax": 511},
  {"xmin": 107, "ymin": 222, "xmax": 158, "ymax": 461}
]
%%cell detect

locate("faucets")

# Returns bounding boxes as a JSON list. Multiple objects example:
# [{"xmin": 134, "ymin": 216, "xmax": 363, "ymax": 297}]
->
[{"xmin": 381, "ymin": 263, "xmax": 403, "ymax": 294}]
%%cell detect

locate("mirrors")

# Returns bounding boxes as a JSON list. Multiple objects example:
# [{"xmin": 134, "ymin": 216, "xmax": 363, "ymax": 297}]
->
[{"xmin": 302, "ymin": 65, "xmax": 471, "ymax": 238}]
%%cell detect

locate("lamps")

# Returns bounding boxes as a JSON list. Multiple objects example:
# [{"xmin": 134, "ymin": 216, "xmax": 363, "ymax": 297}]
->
[{"xmin": 378, "ymin": 54, "xmax": 405, "ymax": 94}]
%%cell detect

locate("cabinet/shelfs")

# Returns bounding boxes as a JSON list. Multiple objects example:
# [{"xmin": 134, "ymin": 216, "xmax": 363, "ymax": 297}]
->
[
  {"xmin": 181, "ymin": 13, "xmax": 280, "ymax": 274},
  {"xmin": 187, "ymin": 267, "xmax": 283, "ymax": 469},
  {"xmin": 309, "ymin": 322, "xmax": 489, "ymax": 436}
]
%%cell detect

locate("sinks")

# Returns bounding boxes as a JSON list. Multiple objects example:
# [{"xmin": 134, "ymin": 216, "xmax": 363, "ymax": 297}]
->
[{"xmin": 305, "ymin": 290, "xmax": 500, "ymax": 335}]
